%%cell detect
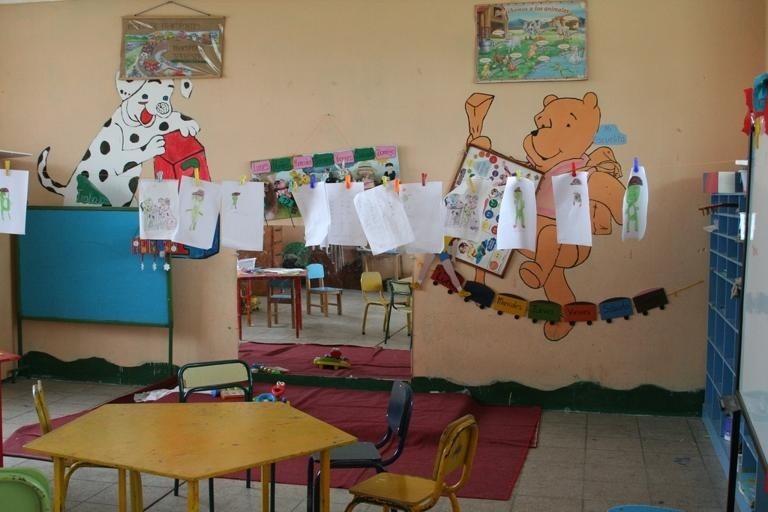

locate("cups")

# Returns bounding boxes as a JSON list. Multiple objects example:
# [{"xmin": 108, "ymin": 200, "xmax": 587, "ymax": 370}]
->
[{"xmin": 718, "ymin": 171, "xmax": 736, "ymax": 195}]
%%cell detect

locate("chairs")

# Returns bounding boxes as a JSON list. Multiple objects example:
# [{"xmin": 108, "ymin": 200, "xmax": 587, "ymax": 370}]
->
[
  {"xmin": 33, "ymin": 381, "xmax": 144, "ymax": 512},
  {"xmin": 175, "ymin": 361, "xmax": 254, "ymax": 494},
  {"xmin": 359, "ymin": 272, "xmax": 411, "ymax": 336},
  {"xmin": 267, "ymin": 278, "xmax": 296, "ymax": 329},
  {"xmin": 1, "ymin": 466, "xmax": 50, "ymax": 512},
  {"xmin": 345, "ymin": 414, "xmax": 478, "ymax": 512},
  {"xmin": 311, "ymin": 381, "xmax": 416, "ymax": 511},
  {"xmin": 307, "ymin": 263, "xmax": 343, "ymax": 316}
]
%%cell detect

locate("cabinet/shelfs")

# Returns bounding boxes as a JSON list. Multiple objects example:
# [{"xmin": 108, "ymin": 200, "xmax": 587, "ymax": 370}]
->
[{"xmin": 704, "ymin": 191, "xmax": 768, "ymax": 512}]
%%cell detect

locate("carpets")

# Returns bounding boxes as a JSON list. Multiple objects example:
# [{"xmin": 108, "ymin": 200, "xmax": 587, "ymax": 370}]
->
[{"xmin": 2, "ymin": 340, "xmax": 539, "ymax": 501}]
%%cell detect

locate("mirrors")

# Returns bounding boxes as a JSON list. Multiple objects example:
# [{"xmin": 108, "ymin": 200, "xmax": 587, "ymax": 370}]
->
[{"xmin": 237, "ymin": 222, "xmax": 414, "ymax": 380}]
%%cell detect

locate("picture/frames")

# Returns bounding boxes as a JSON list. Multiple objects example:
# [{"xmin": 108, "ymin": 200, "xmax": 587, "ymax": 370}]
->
[
  {"xmin": 474, "ymin": 2, "xmax": 588, "ymax": 84},
  {"xmin": 435, "ymin": 146, "xmax": 546, "ymax": 278},
  {"xmin": 122, "ymin": 16, "xmax": 226, "ymax": 78}
]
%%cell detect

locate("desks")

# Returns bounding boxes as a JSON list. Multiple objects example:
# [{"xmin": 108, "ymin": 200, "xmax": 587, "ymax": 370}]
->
[
  {"xmin": 238, "ymin": 270, "xmax": 307, "ymax": 339},
  {"xmin": 385, "ymin": 276, "xmax": 413, "ymax": 344},
  {"xmin": 22, "ymin": 403, "xmax": 358, "ymax": 512},
  {"xmin": 1, "ymin": 348, "xmax": 23, "ymax": 469}
]
